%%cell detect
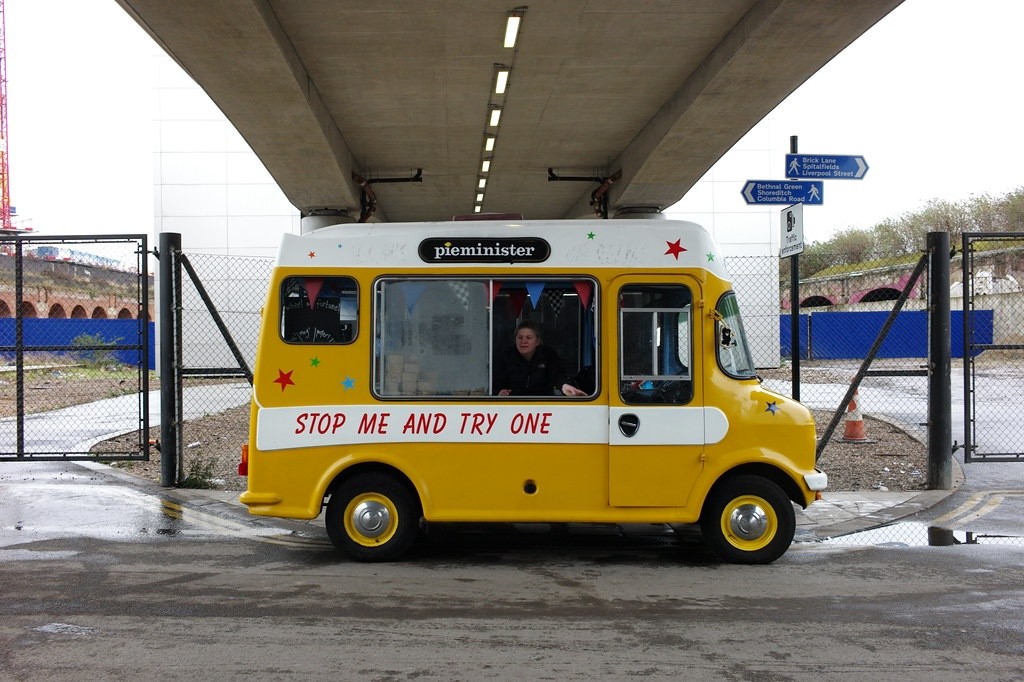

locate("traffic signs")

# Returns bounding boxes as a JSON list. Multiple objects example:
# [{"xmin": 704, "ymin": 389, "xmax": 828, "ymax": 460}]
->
[
  {"xmin": 785, "ymin": 154, "xmax": 870, "ymax": 180},
  {"xmin": 740, "ymin": 179, "xmax": 825, "ymax": 205}
]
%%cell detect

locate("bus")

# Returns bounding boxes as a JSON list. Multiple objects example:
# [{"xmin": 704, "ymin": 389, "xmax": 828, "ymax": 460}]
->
[{"xmin": 238, "ymin": 219, "xmax": 828, "ymax": 567}]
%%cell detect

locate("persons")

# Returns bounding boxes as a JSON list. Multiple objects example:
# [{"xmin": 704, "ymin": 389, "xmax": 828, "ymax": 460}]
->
[{"xmin": 494, "ymin": 321, "xmax": 587, "ymax": 396}]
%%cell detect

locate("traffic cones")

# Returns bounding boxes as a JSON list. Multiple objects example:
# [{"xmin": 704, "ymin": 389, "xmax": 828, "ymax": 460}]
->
[{"xmin": 834, "ymin": 378, "xmax": 878, "ymax": 443}]
